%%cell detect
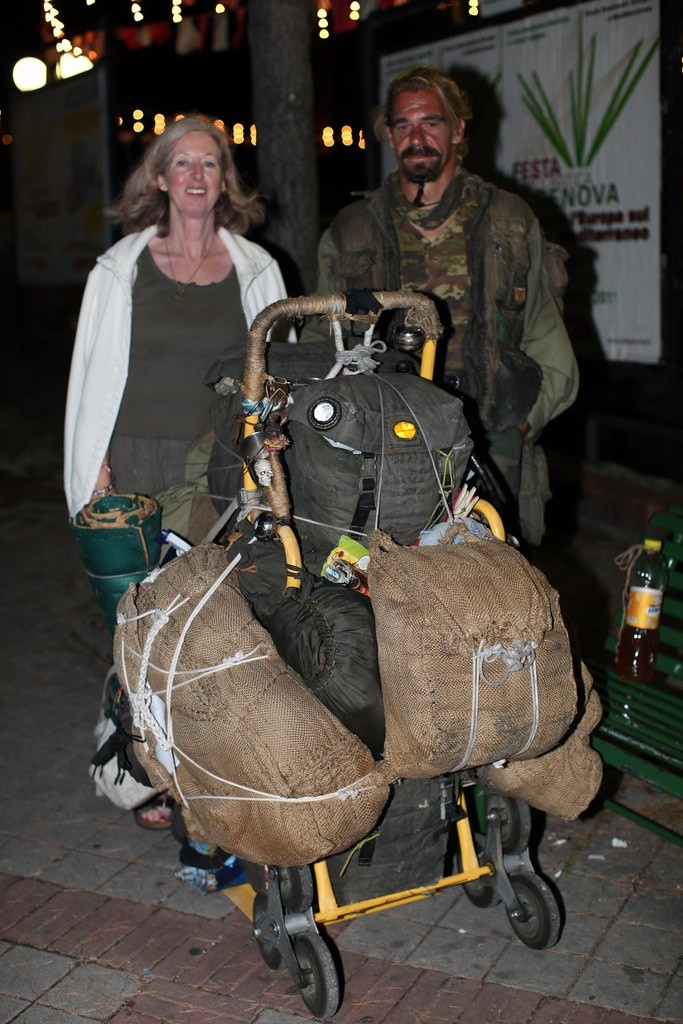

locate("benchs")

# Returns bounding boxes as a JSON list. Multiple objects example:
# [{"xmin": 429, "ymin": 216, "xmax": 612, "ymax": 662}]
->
[{"xmin": 565, "ymin": 502, "xmax": 683, "ymax": 853}]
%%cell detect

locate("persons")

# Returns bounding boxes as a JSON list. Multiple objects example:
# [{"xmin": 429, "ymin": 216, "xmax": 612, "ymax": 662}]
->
[
  {"xmin": 61, "ymin": 114, "xmax": 298, "ymax": 831},
  {"xmin": 300, "ymin": 65, "xmax": 580, "ymax": 570}
]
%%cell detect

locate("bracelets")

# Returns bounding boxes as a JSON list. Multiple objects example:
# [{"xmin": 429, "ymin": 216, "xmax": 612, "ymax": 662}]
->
[
  {"xmin": 93, "ymin": 483, "xmax": 114, "ymax": 499},
  {"xmin": 101, "ymin": 464, "xmax": 111, "ymax": 474}
]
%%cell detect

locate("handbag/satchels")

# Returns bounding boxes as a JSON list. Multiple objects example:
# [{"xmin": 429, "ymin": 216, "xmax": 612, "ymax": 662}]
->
[{"xmin": 94, "ymin": 341, "xmax": 605, "ymax": 869}]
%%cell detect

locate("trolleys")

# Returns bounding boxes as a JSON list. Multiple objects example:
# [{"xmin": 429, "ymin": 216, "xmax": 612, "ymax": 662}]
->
[{"xmin": 115, "ymin": 284, "xmax": 567, "ymax": 1023}]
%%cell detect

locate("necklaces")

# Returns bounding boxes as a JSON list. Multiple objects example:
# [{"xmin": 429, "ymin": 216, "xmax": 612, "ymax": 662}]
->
[{"xmin": 163, "ymin": 231, "xmax": 217, "ymax": 299}]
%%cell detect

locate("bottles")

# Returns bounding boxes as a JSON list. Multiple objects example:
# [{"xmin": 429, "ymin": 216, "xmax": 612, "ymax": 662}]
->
[{"xmin": 614, "ymin": 537, "xmax": 667, "ymax": 684}]
